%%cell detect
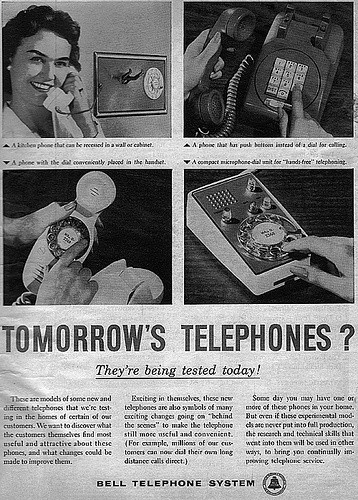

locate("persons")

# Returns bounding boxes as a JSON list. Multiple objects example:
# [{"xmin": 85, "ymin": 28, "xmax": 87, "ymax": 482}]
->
[
  {"xmin": 3, "ymin": 198, "xmax": 98, "ymax": 305},
  {"xmin": 183, "ymin": 28, "xmax": 338, "ymax": 138},
  {"xmin": 281, "ymin": 233, "xmax": 354, "ymax": 302},
  {"xmin": 2, "ymin": 4, "xmax": 104, "ymax": 136}
]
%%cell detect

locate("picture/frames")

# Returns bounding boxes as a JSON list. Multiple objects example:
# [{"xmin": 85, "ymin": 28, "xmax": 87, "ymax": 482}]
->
[{"xmin": 95, "ymin": 51, "xmax": 167, "ymax": 117}]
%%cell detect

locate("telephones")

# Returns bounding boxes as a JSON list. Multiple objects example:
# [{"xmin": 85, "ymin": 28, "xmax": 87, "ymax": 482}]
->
[
  {"xmin": 23, "ymin": 171, "xmax": 117, "ymax": 296},
  {"xmin": 186, "ymin": 171, "xmax": 310, "ymax": 295},
  {"xmin": 42, "ymin": 65, "xmax": 78, "ymax": 113},
  {"xmin": 186, "ymin": 6, "xmax": 343, "ymax": 138}
]
